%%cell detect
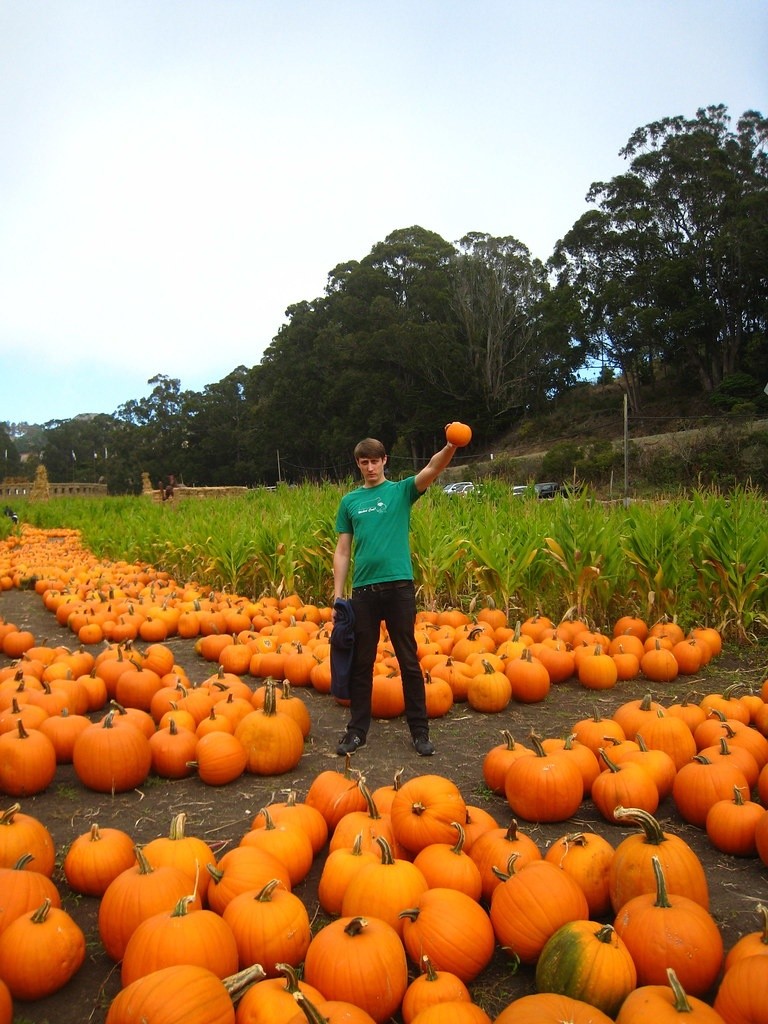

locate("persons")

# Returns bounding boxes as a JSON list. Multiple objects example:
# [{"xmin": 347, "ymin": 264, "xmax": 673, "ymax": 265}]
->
[
  {"xmin": 4, "ymin": 506, "xmax": 18, "ymax": 525},
  {"xmin": 162, "ymin": 474, "xmax": 179, "ymax": 501},
  {"xmin": 331, "ymin": 422, "xmax": 461, "ymax": 755}
]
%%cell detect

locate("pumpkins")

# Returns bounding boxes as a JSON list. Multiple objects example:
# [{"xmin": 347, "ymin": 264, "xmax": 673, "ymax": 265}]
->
[
  {"xmin": 0, "ymin": 521, "xmax": 768, "ymax": 1024},
  {"xmin": 446, "ymin": 423, "xmax": 472, "ymax": 447}
]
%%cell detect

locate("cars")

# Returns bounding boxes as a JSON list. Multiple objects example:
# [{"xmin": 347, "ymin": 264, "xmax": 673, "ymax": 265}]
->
[
  {"xmin": 462, "ymin": 484, "xmax": 486, "ymax": 499},
  {"xmin": 560, "ymin": 486, "xmax": 592, "ymax": 498},
  {"xmin": 443, "ymin": 485, "xmax": 453, "ymax": 493},
  {"xmin": 447, "ymin": 482, "xmax": 473, "ymax": 495},
  {"xmin": 534, "ymin": 482, "xmax": 560, "ymax": 498},
  {"xmin": 511, "ymin": 486, "xmax": 539, "ymax": 496}
]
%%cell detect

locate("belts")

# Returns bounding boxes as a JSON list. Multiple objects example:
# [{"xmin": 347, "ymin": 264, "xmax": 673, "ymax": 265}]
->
[{"xmin": 352, "ymin": 579, "xmax": 412, "ymax": 594}]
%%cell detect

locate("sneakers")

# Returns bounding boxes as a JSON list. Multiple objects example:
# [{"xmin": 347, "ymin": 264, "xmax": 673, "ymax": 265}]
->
[
  {"xmin": 412, "ymin": 731, "xmax": 436, "ymax": 756},
  {"xmin": 337, "ymin": 729, "xmax": 367, "ymax": 756}
]
